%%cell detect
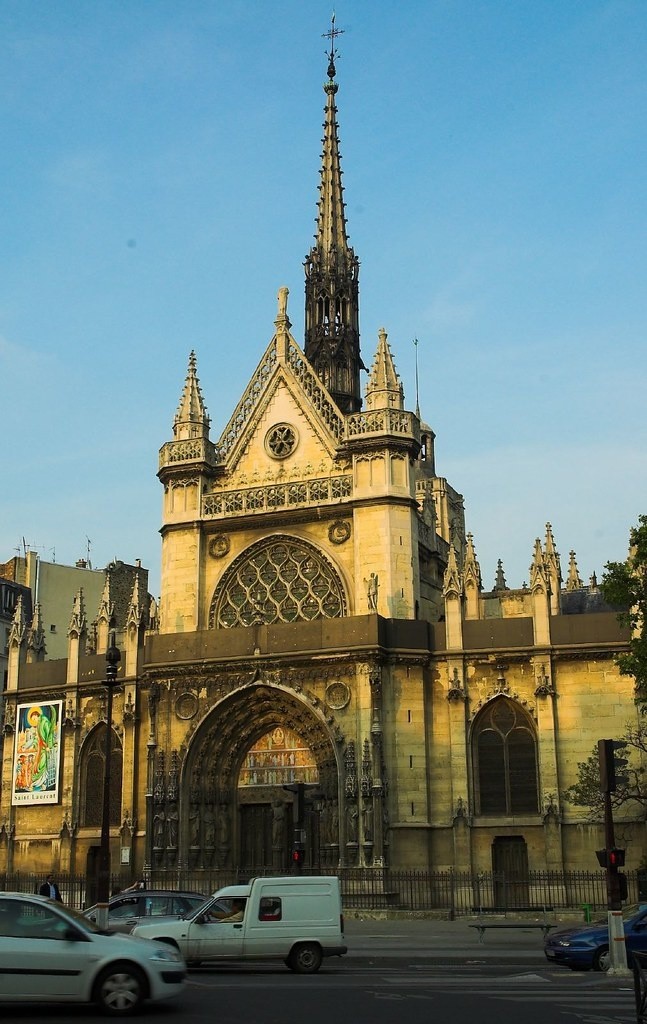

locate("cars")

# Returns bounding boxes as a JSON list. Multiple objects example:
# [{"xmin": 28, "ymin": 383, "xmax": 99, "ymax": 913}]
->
[
  {"xmin": 77, "ymin": 892, "xmax": 233, "ymax": 935},
  {"xmin": 543, "ymin": 901, "xmax": 647, "ymax": 973},
  {"xmin": 1, "ymin": 892, "xmax": 186, "ymax": 1015}
]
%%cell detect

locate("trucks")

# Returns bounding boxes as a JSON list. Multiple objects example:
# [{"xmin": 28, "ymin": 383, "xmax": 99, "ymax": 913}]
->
[{"xmin": 131, "ymin": 876, "xmax": 349, "ymax": 975}]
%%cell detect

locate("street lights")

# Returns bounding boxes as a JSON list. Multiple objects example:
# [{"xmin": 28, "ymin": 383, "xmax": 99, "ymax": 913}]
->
[{"xmin": 99, "ymin": 632, "xmax": 123, "ymax": 901}]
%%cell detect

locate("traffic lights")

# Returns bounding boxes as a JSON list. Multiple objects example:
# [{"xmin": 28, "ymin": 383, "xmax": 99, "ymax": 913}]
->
[
  {"xmin": 303, "ymin": 784, "xmax": 317, "ymax": 808},
  {"xmin": 283, "ymin": 784, "xmax": 300, "ymax": 822},
  {"xmin": 609, "ymin": 738, "xmax": 630, "ymax": 792},
  {"xmin": 608, "ymin": 848, "xmax": 625, "ymax": 867},
  {"xmin": 291, "ymin": 848, "xmax": 302, "ymax": 863},
  {"xmin": 594, "ymin": 850, "xmax": 606, "ymax": 867}
]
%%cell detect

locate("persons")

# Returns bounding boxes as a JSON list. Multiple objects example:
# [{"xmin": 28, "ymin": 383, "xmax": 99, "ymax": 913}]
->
[
  {"xmin": 39, "ymin": 874, "xmax": 64, "ymax": 904},
  {"xmin": 209, "ymin": 899, "xmax": 244, "ymax": 922},
  {"xmin": 134, "ymin": 879, "xmax": 146, "ymax": 893},
  {"xmin": 112, "ymin": 880, "xmax": 137, "ymax": 897},
  {"xmin": 150, "ymin": 596, "xmax": 157, "ymax": 630},
  {"xmin": 367, "ymin": 573, "xmax": 378, "ymax": 614}
]
